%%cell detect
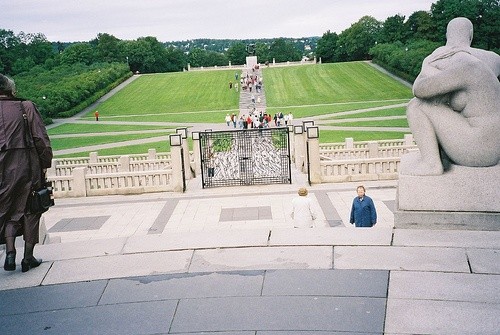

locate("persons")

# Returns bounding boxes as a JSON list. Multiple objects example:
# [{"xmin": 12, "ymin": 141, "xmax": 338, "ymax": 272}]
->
[
  {"xmin": 396, "ymin": 44, "xmax": 500, "ymax": 176},
  {"xmin": 234, "ymin": 64, "xmax": 263, "ymax": 103},
  {"xmin": 229, "ymin": 81, "xmax": 233, "ymax": 89},
  {"xmin": 0, "ymin": 73, "xmax": 54, "ymax": 272},
  {"xmin": 350, "ymin": 185, "xmax": 377, "ymax": 227},
  {"xmin": 225, "ymin": 113, "xmax": 231, "ymax": 126},
  {"xmin": 231, "ymin": 107, "xmax": 294, "ymax": 130},
  {"xmin": 95, "ymin": 110, "xmax": 99, "ymax": 121},
  {"xmin": 422, "ymin": 17, "xmax": 500, "ymax": 79}
]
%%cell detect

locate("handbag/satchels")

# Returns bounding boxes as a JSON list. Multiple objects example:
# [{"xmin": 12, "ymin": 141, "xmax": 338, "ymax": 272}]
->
[{"xmin": 31, "ymin": 185, "xmax": 54, "ymax": 215}]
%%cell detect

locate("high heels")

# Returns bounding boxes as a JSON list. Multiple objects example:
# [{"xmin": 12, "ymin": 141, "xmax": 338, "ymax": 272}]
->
[
  {"xmin": 21, "ymin": 256, "xmax": 42, "ymax": 272},
  {"xmin": 4, "ymin": 253, "xmax": 16, "ymax": 271}
]
